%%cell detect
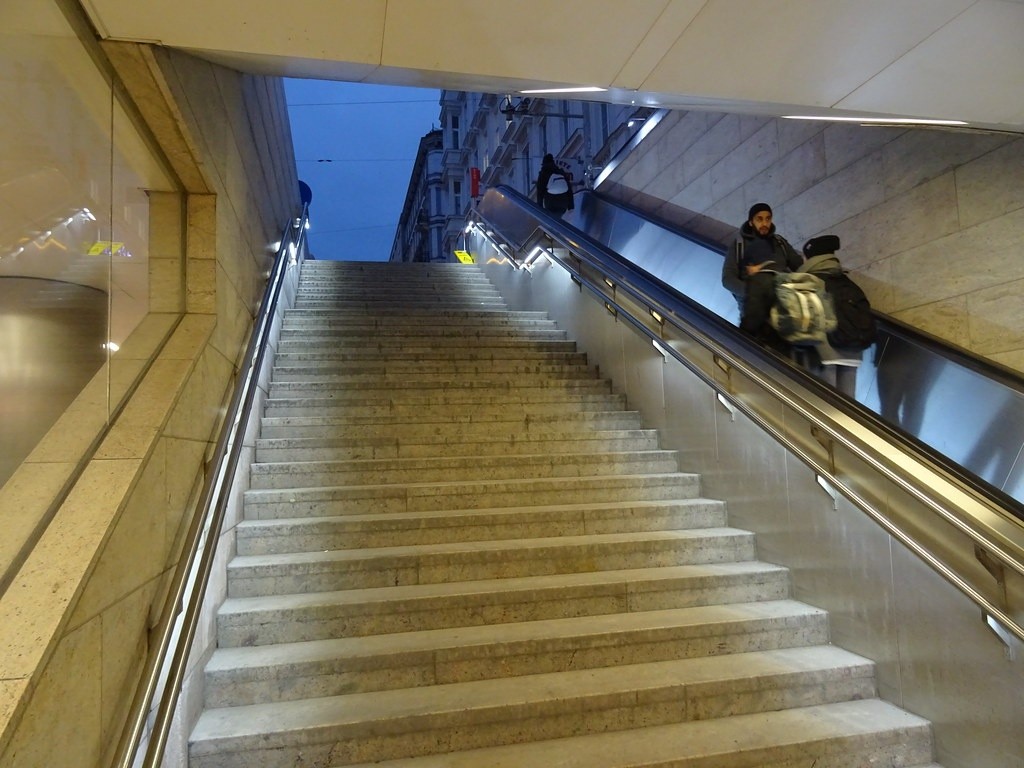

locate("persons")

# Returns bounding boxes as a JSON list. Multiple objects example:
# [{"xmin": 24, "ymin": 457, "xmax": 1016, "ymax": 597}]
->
[
  {"xmin": 536, "ymin": 153, "xmax": 574, "ymax": 219},
  {"xmin": 721, "ymin": 202, "xmax": 875, "ymax": 399}
]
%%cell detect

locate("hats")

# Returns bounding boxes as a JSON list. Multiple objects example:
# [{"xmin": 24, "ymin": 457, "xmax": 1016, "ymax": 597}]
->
[
  {"xmin": 802, "ymin": 234, "xmax": 843, "ymax": 255},
  {"xmin": 748, "ymin": 203, "xmax": 772, "ymax": 216},
  {"xmin": 542, "ymin": 153, "xmax": 555, "ymax": 165}
]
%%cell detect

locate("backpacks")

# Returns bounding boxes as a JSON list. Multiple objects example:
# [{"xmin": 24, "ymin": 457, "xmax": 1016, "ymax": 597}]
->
[
  {"xmin": 546, "ymin": 173, "xmax": 569, "ymax": 195},
  {"xmin": 746, "ymin": 269, "xmax": 838, "ymax": 348}
]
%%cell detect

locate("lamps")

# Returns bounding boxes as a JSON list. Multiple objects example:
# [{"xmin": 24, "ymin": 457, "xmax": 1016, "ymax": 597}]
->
[{"xmin": 627, "ymin": 118, "xmax": 645, "ymax": 127}]
